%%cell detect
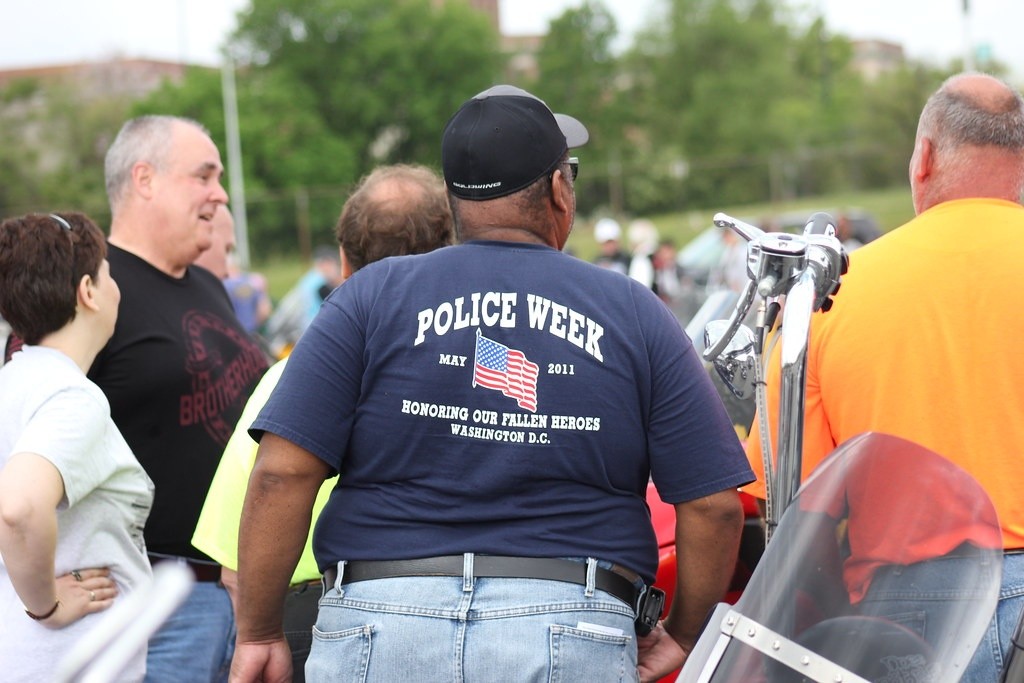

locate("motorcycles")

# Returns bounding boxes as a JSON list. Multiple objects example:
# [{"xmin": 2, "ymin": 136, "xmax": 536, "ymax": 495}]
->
[{"xmin": 625, "ymin": 213, "xmax": 1004, "ymax": 683}]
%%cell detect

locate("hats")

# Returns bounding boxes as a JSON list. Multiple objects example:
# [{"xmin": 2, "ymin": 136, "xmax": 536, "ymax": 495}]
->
[
  {"xmin": 594, "ymin": 219, "xmax": 621, "ymax": 242},
  {"xmin": 441, "ymin": 84, "xmax": 589, "ymax": 201}
]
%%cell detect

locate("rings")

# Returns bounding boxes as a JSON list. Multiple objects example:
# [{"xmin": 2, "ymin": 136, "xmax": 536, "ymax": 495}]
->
[
  {"xmin": 70, "ymin": 569, "xmax": 83, "ymax": 581},
  {"xmin": 89, "ymin": 591, "xmax": 95, "ymax": 601}
]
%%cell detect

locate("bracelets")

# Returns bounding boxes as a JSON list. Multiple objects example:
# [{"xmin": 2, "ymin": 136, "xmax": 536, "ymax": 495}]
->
[{"xmin": 23, "ymin": 600, "xmax": 59, "ymax": 620}]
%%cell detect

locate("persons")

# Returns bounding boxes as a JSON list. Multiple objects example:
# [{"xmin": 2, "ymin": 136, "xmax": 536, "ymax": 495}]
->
[
  {"xmin": 0, "ymin": 209, "xmax": 158, "ymax": 683},
  {"xmin": 193, "ymin": 163, "xmax": 455, "ymax": 683},
  {"xmin": 738, "ymin": 68, "xmax": 1023, "ymax": 678},
  {"xmin": 233, "ymin": 209, "xmax": 870, "ymax": 409},
  {"xmin": 233, "ymin": 88, "xmax": 758, "ymax": 683},
  {"xmin": 192, "ymin": 199, "xmax": 236, "ymax": 284},
  {"xmin": 77, "ymin": 118, "xmax": 269, "ymax": 683}
]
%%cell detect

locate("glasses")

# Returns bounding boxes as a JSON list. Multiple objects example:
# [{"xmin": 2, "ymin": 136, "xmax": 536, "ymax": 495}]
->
[
  {"xmin": 549, "ymin": 157, "xmax": 579, "ymax": 180},
  {"xmin": 49, "ymin": 213, "xmax": 78, "ymax": 307}
]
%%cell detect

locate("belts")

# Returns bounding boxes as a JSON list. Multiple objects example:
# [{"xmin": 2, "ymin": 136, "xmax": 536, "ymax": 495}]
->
[
  {"xmin": 322, "ymin": 553, "xmax": 640, "ymax": 613},
  {"xmin": 933, "ymin": 544, "xmax": 1024, "ymax": 560},
  {"xmin": 147, "ymin": 552, "xmax": 222, "ymax": 582}
]
